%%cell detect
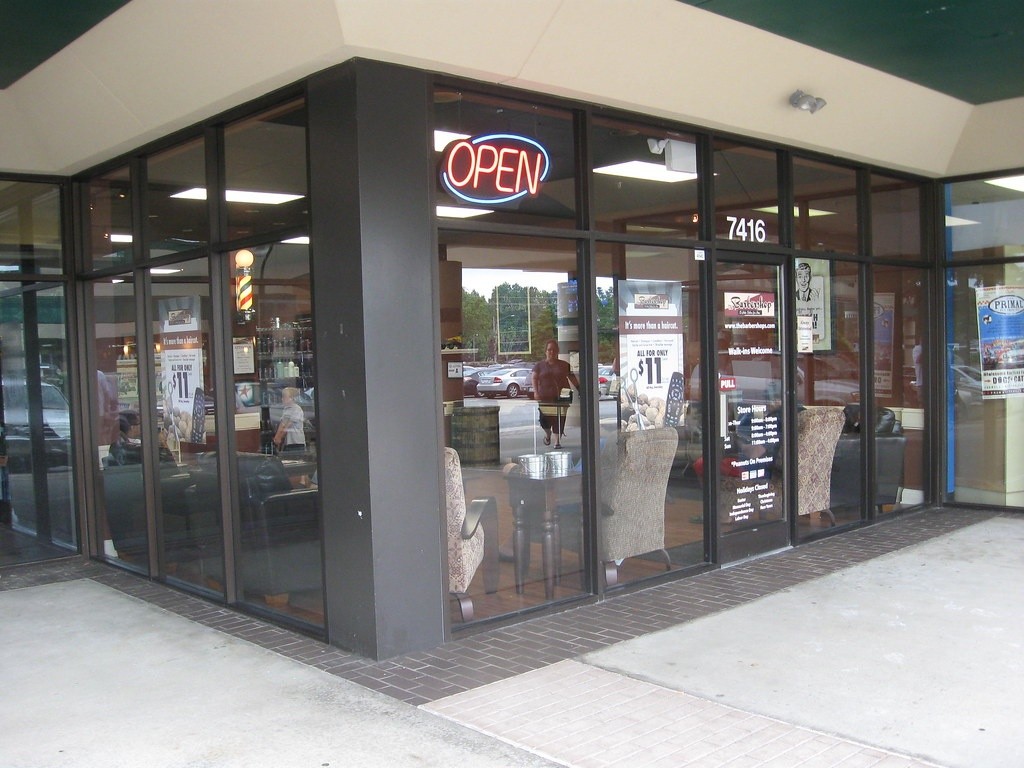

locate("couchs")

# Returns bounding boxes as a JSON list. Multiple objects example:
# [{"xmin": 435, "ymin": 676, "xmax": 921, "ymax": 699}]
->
[
  {"xmin": 720, "ymin": 406, "xmax": 847, "ymax": 528},
  {"xmin": 531, "ymin": 425, "xmax": 679, "ymax": 585},
  {"xmin": 185, "ymin": 450, "xmax": 324, "ymax": 607},
  {"xmin": 445, "ymin": 446, "xmax": 500, "ymax": 622},
  {"xmin": 99, "ymin": 456, "xmax": 197, "ymax": 554},
  {"xmin": 828, "ymin": 404, "xmax": 908, "ymax": 515}
]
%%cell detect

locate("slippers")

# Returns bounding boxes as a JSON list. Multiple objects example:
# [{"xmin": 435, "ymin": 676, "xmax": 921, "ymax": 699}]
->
[
  {"xmin": 553, "ymin": 442, "xmax": 561, "ymax": 449},
  {"xmin": 543, "ymin": 431, "xmax": 552, "ymax": 445}
]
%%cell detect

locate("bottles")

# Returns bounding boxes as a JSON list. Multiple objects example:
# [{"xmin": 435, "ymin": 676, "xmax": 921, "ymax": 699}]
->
[
  {"xmin": 262, "ymin": 387, "xmax": 308, "ymax": 405},
  {"xmin": 263, "ymin": 337, "xmax": 311, "ymax": 351},
  {"xmin": 276, "ymin": 316, "xmax": 280, "ymax": 328},
  {"xmin": 257, "ymin": 338, "xmax": 261, "ymax": 352},
  {"xmin": 258, "ymin": 361, "xmax": 316, "ymax": 378}
]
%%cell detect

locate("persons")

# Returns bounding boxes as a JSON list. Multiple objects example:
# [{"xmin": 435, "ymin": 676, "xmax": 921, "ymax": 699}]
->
[
  {"xmin": 107, "ymin": 408, "xmax": 141, "ymax": 465},
  {"xmin": 688, "ymin": 380, "xmax": 781, "ymax": 526},
  {"xmin": 273, "ymin": 386, "xmax": 306, "ymax": 486},
  {"xmin": 531, "ymin": 339, "xmax": 581, "ymax": 447},
  {"xmin": 912, "ymin": 333, "xmax": 924, "ymax": 410},
  {"xmin": 96, "ymin": 368, "xmax": 111, "ymax": 442}
]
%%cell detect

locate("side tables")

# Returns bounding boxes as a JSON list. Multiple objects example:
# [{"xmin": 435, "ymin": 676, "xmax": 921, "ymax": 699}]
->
[{"xmin": 503, "ymin": 470, "xmax": 584, "ymax": 600}]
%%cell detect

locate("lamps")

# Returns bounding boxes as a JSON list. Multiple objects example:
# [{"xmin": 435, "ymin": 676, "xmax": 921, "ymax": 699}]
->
[{"xmin": 234, "ymin": 250, "xmax": 256, "ymax": 325}]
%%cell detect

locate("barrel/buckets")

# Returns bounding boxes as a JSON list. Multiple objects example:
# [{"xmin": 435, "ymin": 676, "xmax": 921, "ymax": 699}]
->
[
  {"xmin": 546, "ymin": 452, "xmax": 571, "ymax": 473},
  {"xmin": 518, "ymin": 454, "xmax": 547, "ymax": 472},
  {"xmin": 451, "ymin": 407, "xmax": 500, "ymax": 466}
]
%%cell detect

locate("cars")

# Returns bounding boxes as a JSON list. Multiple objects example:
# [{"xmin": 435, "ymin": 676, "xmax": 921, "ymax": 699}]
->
[
  {"xmin": 1, "ymin": 375, "xmax": 71, "ymax": 456},
  {"xmin": 477, "ymin": 368, "xmax": 533, "ymax": 399},
  {"xmin": 902, "ymin": 365, "xmax": 983, "ymax": 407},
  {"xmin": 233, "ymin": 382, "xmax": 318, "ymax": 448},
  {"xmin": 521, "ymin": 371, "xmax": 534, "ymax": 400},
  {"xmin": 156, "ymin": 395, "xmax": 215, "ymax": 416},
  {"xmin": 598, "ymin": 366, "xmax": 618, "ymax": 401},
  {"xmin": 489, "ymin": 358, "xmax": 539, "ymax": 369},
  {"xmin": 463, "ymin": 368, "xmax": 498, "ymax": 398}
]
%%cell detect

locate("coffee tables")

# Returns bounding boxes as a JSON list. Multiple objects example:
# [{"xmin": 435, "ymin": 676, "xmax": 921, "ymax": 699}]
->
[{"xmin": 278, "ymin": 458, "xmax": 316, "ymax": 475}]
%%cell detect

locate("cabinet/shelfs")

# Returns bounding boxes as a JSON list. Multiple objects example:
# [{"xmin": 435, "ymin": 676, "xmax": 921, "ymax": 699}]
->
[{"xmin": 256, "ymin": 326, "xmax": 316, "ymax": 454}]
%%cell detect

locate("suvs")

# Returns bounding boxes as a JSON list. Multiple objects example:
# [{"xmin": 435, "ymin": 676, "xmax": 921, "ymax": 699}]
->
[{"xmin": 689, "ymin": 352, "xmax": 860, "ymax": 432}]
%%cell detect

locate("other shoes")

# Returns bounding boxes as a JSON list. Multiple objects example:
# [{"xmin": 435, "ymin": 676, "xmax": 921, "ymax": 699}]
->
[{"xmin": 689, "ymin": 512, "xmax": 705, "ymax": 523}]
